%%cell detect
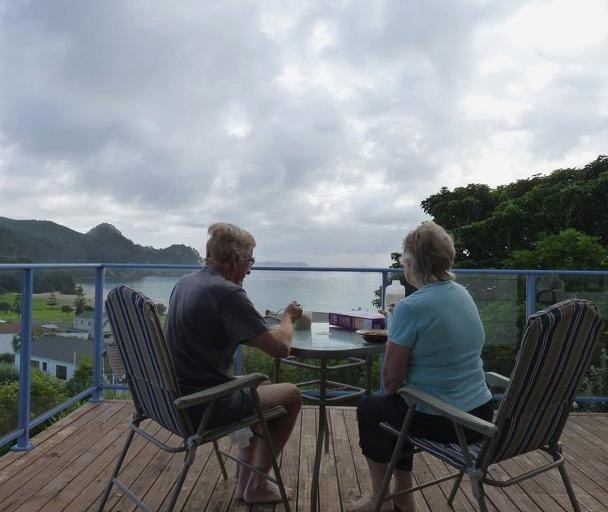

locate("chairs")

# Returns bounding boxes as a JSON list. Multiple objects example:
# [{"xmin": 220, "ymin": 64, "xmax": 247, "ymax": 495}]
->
[
  {"xmin": 369, "ymin": 297, "xmax": 607, "ymax": 511},
  {"xmin": 97, "ymin": 287, "xmax": 292, "ymax": 512}
]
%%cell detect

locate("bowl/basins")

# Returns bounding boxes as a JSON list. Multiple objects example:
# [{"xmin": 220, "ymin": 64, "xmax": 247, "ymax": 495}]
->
[{"xmin": 294, "ymin": 310, "xmax": 313, "ymax": 330}]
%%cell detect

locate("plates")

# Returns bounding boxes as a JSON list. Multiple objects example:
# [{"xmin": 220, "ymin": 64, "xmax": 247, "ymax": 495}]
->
[{"xmin": 354, "ymin": 328, "xmax": 389, "ymax": 343}]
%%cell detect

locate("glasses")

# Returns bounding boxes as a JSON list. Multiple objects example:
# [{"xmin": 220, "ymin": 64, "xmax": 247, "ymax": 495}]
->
[{"xmin": 245, "ymin": 257, "xmax": 255, "ymax": 267}]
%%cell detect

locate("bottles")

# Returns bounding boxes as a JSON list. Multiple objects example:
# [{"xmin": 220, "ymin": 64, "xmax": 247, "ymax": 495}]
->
[{"xmin": 385, "ymin": 279, "xmax": 406, "ymax": 335}]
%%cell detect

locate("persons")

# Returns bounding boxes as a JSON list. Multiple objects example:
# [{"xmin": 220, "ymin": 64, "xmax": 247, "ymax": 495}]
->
[
  {"xmin": 344, "ymin": 220, "xmax": 494, "ymax": 512},
  {"xmin": 163, "ymin": 222, "xmax": 304, "ymax": 504}
]
